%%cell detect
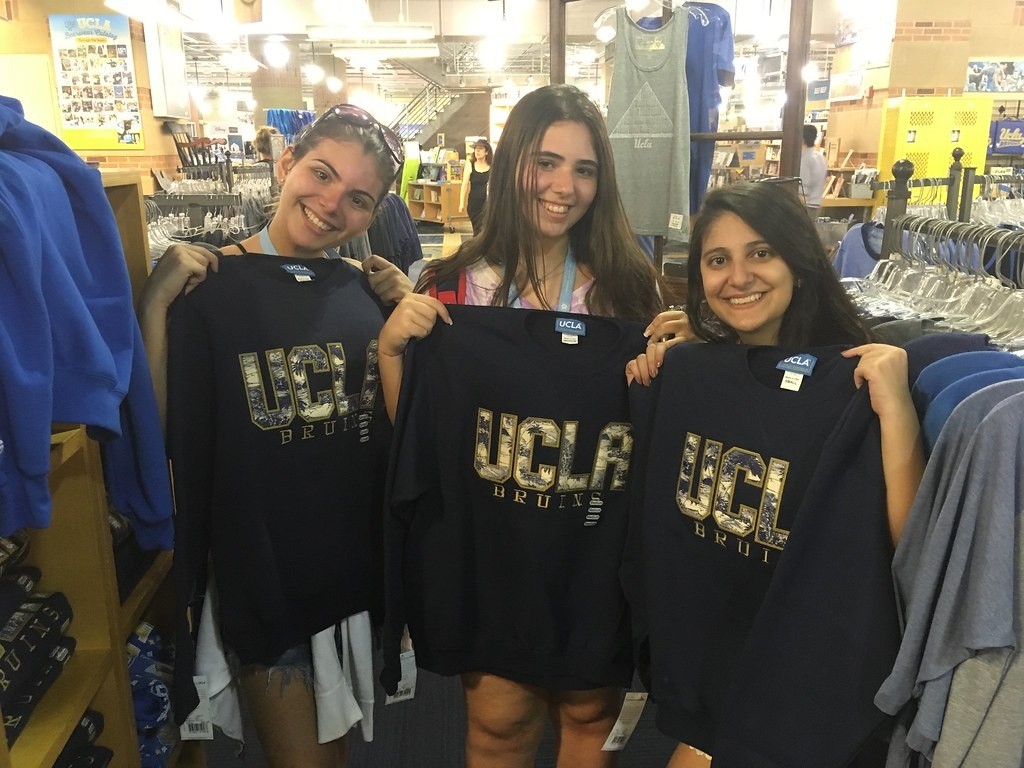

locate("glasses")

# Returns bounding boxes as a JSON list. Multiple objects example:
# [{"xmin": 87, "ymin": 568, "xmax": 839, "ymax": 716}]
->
[
  {"xmin": 731, "ymin": 175, "xmax": 807, "ymax": 214},
  {"xmin": 299, "ymin": 104, "xmax": 405, "ymax": 209}
]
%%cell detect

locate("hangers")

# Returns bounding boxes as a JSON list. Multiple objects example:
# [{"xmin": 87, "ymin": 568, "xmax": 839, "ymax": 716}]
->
[
  {"xmin": 865, "ymin": 173, "xmax": 1024, "ymax": 228},
  {"xmin": 142, "ymin": 165, "xmax": 273, "ymax": 269},
  {"xmin": 593, "ymin": 0, "xmax": 709, "ymax": 27},
  {"xmin": 837, "ymin": 212, "xmax": 1024, "ymax": 361}
]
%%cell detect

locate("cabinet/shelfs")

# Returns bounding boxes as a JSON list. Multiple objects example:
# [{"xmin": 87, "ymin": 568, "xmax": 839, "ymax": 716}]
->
[
  {"xmin": 0, "ymin": 165, "xmax": 174, "ymax": 768},
  {"xmin": 703, "ymin": 144, "xmax": 874, "ymax": 207},
  {"xmin": 408, "ymin": 180, "xmax": 470, "ymax": 233}
]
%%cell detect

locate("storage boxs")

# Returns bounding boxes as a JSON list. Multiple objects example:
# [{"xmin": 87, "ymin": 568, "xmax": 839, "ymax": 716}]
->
[
  {"xmin": 847, "ymin": 183, "xmax": 873, "ymax": 199},
  {"xmin": 814, "ymin": 224, "xmax": 850, "ymax": 250},
  {"xmin": 987, "ymin": 119, "xmax": 1024, "ymax": 155}
]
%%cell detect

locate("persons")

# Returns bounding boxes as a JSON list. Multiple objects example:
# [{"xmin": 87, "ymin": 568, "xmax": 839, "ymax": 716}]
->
[
  {"xmin": 797, "ymin": 124, "xmax": 828, "ymax": 218},
  {"xmin": 626, "ymin": 180, "xmax": 929, "ymax": 768},
  {"xmin": 115, "ymin": 100, "xmax": 125, "ymax": 112},
  {"xmin": 459, "ymin": 138, "xmax": 493, "ymax": 238},
  {"xmin": 377, "ymin": 84, "xmax": 699, "ymax": 768},
  {"xmin": 253, "ymin": 126, "xmax": 280, "ymax": 172},
  {"xmin": 136, "ymin": 112, "xmax": 416, "ymax": 768}
]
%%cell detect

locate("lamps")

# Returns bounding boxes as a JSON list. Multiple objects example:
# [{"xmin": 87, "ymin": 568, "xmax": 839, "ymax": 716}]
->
[
  {"xmin": 190, "ymin": 0, "xmax": 343, "ymax": 102},
  {"xmin": 458, "ymin": 77, "xmax": 536, "ymax": 87}
]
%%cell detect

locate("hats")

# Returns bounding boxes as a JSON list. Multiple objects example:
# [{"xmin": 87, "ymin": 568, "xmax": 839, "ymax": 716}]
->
[{"xmin": 470, "ymin": 139, "xmax": 490, "ymax": 151}]
